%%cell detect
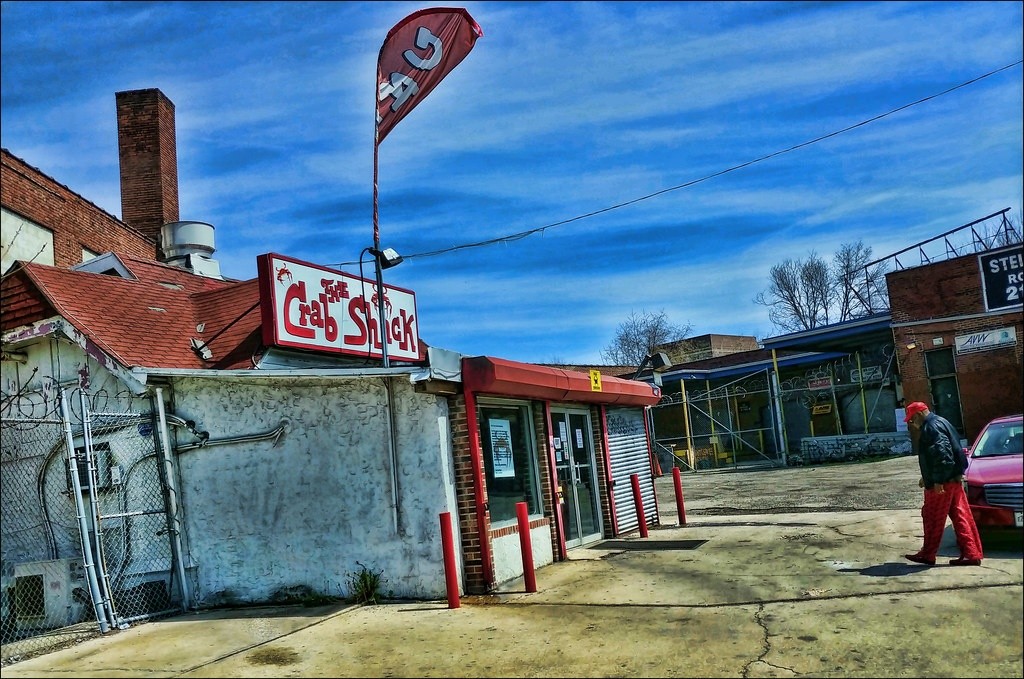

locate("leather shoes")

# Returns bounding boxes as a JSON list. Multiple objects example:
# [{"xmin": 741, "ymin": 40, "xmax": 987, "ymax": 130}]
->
[
  {"xmin": 905, "ymin": 554, "xmax": 936, "ymax": 565},
  {"xmin": 949, "ymin": 555, "xmax": 981, "ymax": 566}
]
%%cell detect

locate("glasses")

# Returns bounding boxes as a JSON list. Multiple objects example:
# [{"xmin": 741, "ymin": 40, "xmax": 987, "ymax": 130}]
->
[{"xmin": 909, "ymin": 415, "xmax": 919, "ymax": 423}]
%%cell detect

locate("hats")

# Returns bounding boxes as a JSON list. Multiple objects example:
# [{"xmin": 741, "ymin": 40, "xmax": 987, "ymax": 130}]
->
[{"xmin": 903, "ymin": 402, "xmax": 928, "ymax": 422}]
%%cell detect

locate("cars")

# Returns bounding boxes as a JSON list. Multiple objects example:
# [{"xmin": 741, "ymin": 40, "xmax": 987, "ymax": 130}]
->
[{"xmin": 959, "ymin": 415, "xmax": 1024, "ymax": 548}]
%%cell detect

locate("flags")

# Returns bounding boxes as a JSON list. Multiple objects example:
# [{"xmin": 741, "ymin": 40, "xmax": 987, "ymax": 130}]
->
[{"xmin": 377, "ymin": 6, "xmax": 484, "ymax": 147}]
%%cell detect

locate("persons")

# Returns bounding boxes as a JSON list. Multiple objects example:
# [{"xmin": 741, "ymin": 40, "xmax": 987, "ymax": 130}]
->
[{"xmin": 904, "ymin": 402, "xmax": 985, "ymax": 567}]
